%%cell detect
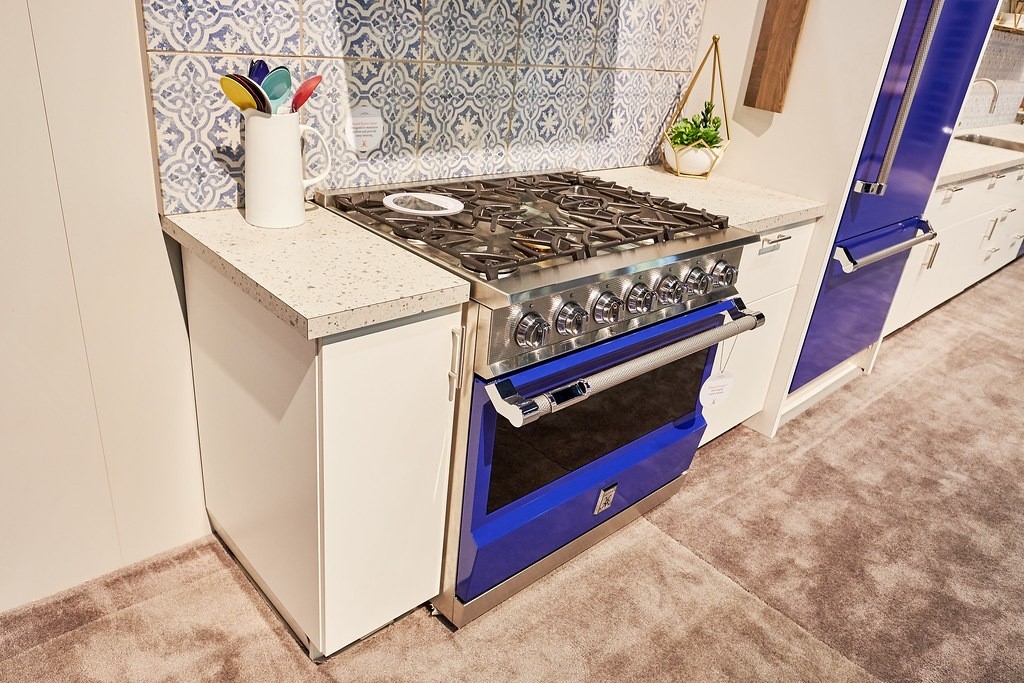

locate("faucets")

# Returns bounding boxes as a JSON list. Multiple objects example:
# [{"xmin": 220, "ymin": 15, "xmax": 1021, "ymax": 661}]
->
[{"xmin": 972, "ymin": 77, "xmax": 1000, "ymax": 115}]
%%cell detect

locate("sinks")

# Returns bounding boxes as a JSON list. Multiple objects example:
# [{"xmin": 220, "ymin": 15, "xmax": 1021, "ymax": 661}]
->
[{"xmin": 954, "ymin": 133, "xmax": 1024, "ymax": 153}]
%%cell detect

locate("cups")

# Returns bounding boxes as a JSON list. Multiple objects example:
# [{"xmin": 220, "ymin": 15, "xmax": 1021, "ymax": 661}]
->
[{"xmin": 235, "ymin": 108, "xmax": 331, "ymax": 228}]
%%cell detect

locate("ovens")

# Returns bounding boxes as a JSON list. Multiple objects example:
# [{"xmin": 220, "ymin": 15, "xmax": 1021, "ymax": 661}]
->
[{"xmin": 439, "ymin": 300, "xmax": 766, "ymax": 628}]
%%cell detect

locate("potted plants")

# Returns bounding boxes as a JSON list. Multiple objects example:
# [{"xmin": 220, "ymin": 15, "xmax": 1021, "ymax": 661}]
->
[{"xmin": 665, "ymin": 102, "xmax": 725, "ymax": 174}]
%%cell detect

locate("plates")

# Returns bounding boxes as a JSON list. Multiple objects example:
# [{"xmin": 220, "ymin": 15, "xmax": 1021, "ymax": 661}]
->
[{"xmin": 383, "ymin": 193, "xmax": 465, "ymax": 216}]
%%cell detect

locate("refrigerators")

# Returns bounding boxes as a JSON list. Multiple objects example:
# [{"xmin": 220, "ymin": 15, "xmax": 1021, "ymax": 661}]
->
[{"xmin": 742, "ymin": 0, "xmax": 1000, "ymax": 437}]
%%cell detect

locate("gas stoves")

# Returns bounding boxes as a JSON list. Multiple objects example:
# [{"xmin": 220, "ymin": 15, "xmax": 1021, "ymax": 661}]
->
[{"xmin": 323, "ymin": 170, "xmax": 760, "ymax": 379}]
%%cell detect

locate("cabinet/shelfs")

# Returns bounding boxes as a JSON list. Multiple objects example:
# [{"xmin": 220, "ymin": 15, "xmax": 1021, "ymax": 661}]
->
[
  {"xmin": 162, "ymin": 201, "xmax": 470, "ymax": 660},
  {"xmin": 880, "ymin": 140, "xmax": 1024, "ymax": 341},
  {"xmin": 580, "ymin": 162, "xmax": 827, "ymax": 449}
]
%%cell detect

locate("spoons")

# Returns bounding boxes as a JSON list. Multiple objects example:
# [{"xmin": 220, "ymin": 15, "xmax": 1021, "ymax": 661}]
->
[{"xmin": 220, "ymin": 61, "xmax": 322, "ymax": 113}]
